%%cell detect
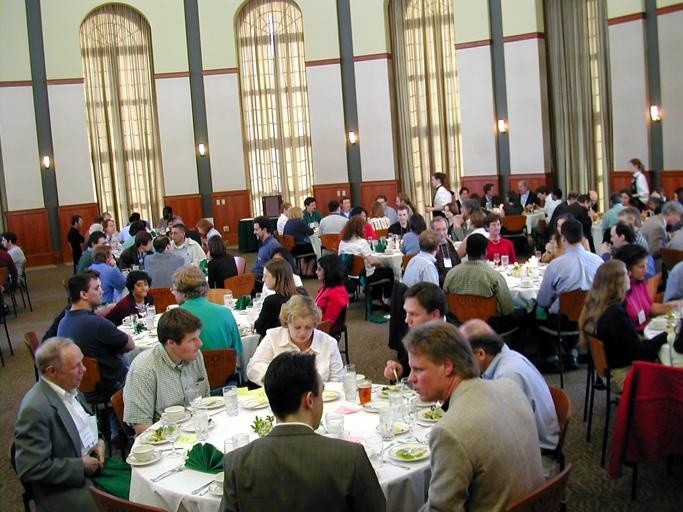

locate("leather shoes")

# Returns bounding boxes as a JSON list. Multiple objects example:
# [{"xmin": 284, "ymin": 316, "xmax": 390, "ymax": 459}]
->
[{"xmin": 569, "ymin": 355, "xmax": 579, "ymax": 369}]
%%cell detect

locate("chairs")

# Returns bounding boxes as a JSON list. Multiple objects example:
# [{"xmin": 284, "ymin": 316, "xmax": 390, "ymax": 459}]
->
[
  {"xmin": 501, "ymin": 215, "xmax": 529, "ymax": 253},
  {"xmin": 541, "ymin": 385, "xmax": 571, "ymax": 509},
  {"xmin": 225, "ymin": 273, "xmax": 257, "ymax": 299},
  {"xmin": 659, "ymin": 246, "xmax": 683, "ymax": 272},
  {"xmin": 645, "ymin": 272, "xmax": 662, "ymax": 301},
  {"xmin": 148, "ymin": 287, "xmax": 178, "ymax": 312},
  {"xmin": 22, "ymin": 330, "xmax": 40, "ymax": 382},
  {"xmin": 628, "ymin": 361, "xmax": 683, "ymax": 501},
  {"xmin": 234, "ymin": 256, "xmax": 248, "ymax": 274},
  {"xmin": 78, "ymin": 358, "xmax": 116, "ymax": 458},
  {"xmin": 320, "ymin": 233, "xmax": 341, "ymax": 256},
  {"xmin": 341, "ymin": 254, "xmax": 390, "ymax": 322},
  {"xmin": 12, "ymin": 257, "xmax": 33, "ymax": 311},
  {"xmin": 332, "ymin": 304, "xmax": 352, "ymax": 364},
  {"xmin": 7, "ymin": 440, "xmax": 35, "ymax": 511},
  {"xmin": 447, "ymin": 292, "xmax": 520, "ymax": 345},
  {"xmin": 505, "ymin": 462, "xmax": 574, "ymax": 512},
  {"xmin": 585, "ymin": 331, "xmax": 621, "ymax": 471},
  {"xmin": 276, "ymin": 233, "xmax": 318, "ymax": 278},
  {"xmin": 110, "ymin": 388, "xmax": 133, "ymax": 461},
  {"xmin": 0, "ymin": 265, "xmax": 18, "ymax": 319},
  {"xmin": 540, "ymin": 289, "xmax": 590, "ymax": 387},
  {"xmin": 88, "ymin": 485, "xmax": 165, "ymax": 512},
  {"xmin": 202, "ymin": 348, "xmax": 238, "ymax": 389}
]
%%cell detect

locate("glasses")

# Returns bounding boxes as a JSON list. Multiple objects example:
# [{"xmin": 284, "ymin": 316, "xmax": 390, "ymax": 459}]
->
[{"xmin": 170, "ymin": 287, "xmax": 178, "ymax": 293}]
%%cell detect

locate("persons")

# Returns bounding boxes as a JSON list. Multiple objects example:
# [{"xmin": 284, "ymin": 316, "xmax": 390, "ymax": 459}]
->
[
  {"xmin": 404, "ymin": 321, "xmax": 544, "ymax": 512},
  {"xmin": 124, "ymin": 309, "xmax": 211, "ymax": 436},
  {"xmin": 368, "ymin": 202, "xmax": 391, "ymax": 229},
  {"xmin": 58, "ymin": 272, "xmax": 134, "ymax": 393},
  {"xmin": 388, "ymin": 205, "xmax": 418, "ymax": 236},
  {"xmin": 456, "ymin": 187, "xmax": 470, "ymax": 214},
  {"xmin": 169, "ymin": 224, "xmax": 206, "ymax": 267},
  {"xmin": 402, "ymin": 229, "xmax": 441, "ymax": 288},
  {"xmin": 262, "ymin": 247, "xmax": 303, "ymax": 299},
  {"xmin": 642, "ymin": 202, "xmax": 683, "ymax": 264},
  {"xmin": 619, "ymin": 189, "xmax": 633, "ymax": 209},
  {"xmin": 339, "ymin": 196, "xmax": 354, "ymax": 217},
  {"xmin": 77, "ymin": 231, "xmax": 107, "ymax": 274},
  {"xmin": 172, "ymin": 267, "xmax": 243, "ymax": 368},
  {"xmin": 630, "ymin": 158, "xmax": 650, "ymax": 212},
  {"xmin": 614, "ymin": 243, "xmax": 678, "ymax": 335},
  {"xmin": 42, "ymin": 269, "xmax": 99, "ymax": 345},
  {"xmin": 443, "ymin": 233, "xmax": 514, "ymax": 315},
  {"xmin": 444, "ymin": 203, "xmax": 460, "ymax": 235},
  {"xmin": 144, "ymin": 235, "xmax": 185, "ymax": 288},
  {"xmin": 602, "ymin": 192, "xmax": 632, "ymax": 233},
  {"xmin": 611, "ymin": 222, "xmax": 656, "ymax": 281},
  {"xmin": 303, "ymin": 198, "xmax": 323, "ymax": 226},
  {"xmin": 480, "ymin": 183, "xmax": 498, "ymax": 211},
  {"xmin": 396, "ymin": 192, "xmax": 416, "ymax": 220},
  {"xmin": 430, "ymin": 218, "xmax": 461, "ymax": 282},
  {"xmin": 460, "ymin": 319, "xmax": 560, "ymax": 454},
  {"xmin": 103, "ymin": 219, "xmax": 122, "ymax": 251},
  {"xmin": 319, "ymin": 200, "xmax": 350, "ymax": 234},
  {"xmin": 88, "ymin": 246, "xmax": 127, "ymax": 313},
  {"xmin": 452, "ymin": 199, "xmax": 485, "ymax": 241},
  {"xmin": 67, "ymin": 216, "xmax": 85, "ymax": 274},
  {"xmin": 117, "ymin": 232, "xmax": 154, "ymax": 270},
  {"xmin": 15, "ymin": 337, "xmax": 106, "ymax": 511},
  {"xmin": 219, "ymin": 351, "xmax": 385, "ymax": 511},
  {"xmin": 385, "ymin": 282, "xmax": 460, "ymax": 384},
  {"xmin": 425, "ymin": 173, "xmax": 452, "ymax": 221},
  {"xmin": 499, "ymin": 190, "xmax": 523, "ymax": 216},
  {"xmin": 2, "ymin": 231, "xmax": 25, "ymax": 283},
  {"xmin": 652, "ymin": 188, "xmax": 666, "ymax": 203},
  {"xmin": 375, "ymin": 194, "xmax": 397, "ymax": 225},
  {"xmin": 0, "ymin": 249, "xmax": 18, "ymax": 313},
  {"xmin": 539, "ymin": 213, "xmax": 591, "ymax": 264},
  {"xmin": 618, "ymin": 208, "xmax": 650, "ymax": 255},
  {"xmin": 314, "ymin": 254, "xmax": 350, "ymax": 334},
  {"xmin": 547, "ymin": 192, "xmax": 580, "ymax": 238},
  {"xmin": 255, "ymin": 258, "xmax": 309, "ymax": 340},
  {"xmin": 198, "ymin": 220, "xmax": 222, "ymax": 248},
  {"xmin": 339, "ymin": 216, "xmax": 394, "ymax": 313},
  {"xmin": 284, "ymin": 207, "xmax": 316, "ymax": 278},
  {"xmin": 546, "ymin": 188, "xmax": 563, "ymax": 223},
  {"xmin": 457, "ymin": 212, "xmax": 489, "ymax": 259},
  {"xmin": 579, "ymin": 259, "xmax": 673, "ymax": 392},
  {"xmin": 253, "ymin": 217, "xmax": 280, "ymax": 273},
  {"xmin": 174, "ymin": 219, "xmax": 202, "ymax": 247},
  {"xmin": 536, "ymin": 219, "xmax": 604, "ymax": 332},
  {"xmin": 277, "ymin": 203, "xmax": 293, "ymax": 235},
  {"xmin": 208, "ymin": 235, "xmax": 238, "ymax": 289},
  {"xmin": 517, "ymin": 180, "xmax": 540, "ymax": 210},
  {"xmin": 103, "ymin": 271, "xmax": 152, "ymax": 324},
  {"xmin": 483, "ymin": 214, "xmax": 517, "ymax": 264},
  {"xmin": 123, "ymin": 221, "xmax": 156, "ymax": 255},
  {"xmin": 118, "ymin": 213, "xmax": 150, "ymax": 243},
  {"xmin": 674, "ymin": 187, "xmax": 683, "ymax": 206},
  {"xmin": 536, "ymin": 185, "xmax": 553, "ymax": 211},
  {"xmin": 349, "ymin": 206, "xmax": 377, "ymax": 239},
  {"xmin": 648, "ymin": 198, "xmax": 665, "ymax": 216},
  {"xmin": 246, "ymin": 294, "xmax": 344, "ymax": 387},
  {"xmin": 561, "ymin": 195, "xmax": 596, "ymax": 254},
  {"xmin": 157, "ymin": 207, "xmax": 182, "ymax": 228},
  {"xmin": 588, "ymin": 191, "xmax": 598, "ymax": 213}
]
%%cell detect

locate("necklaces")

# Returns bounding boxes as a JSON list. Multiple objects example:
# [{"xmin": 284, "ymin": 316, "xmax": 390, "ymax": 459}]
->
[{"xmin": 489, "ymin": 238, "xmax": 500, "ymax": 245}]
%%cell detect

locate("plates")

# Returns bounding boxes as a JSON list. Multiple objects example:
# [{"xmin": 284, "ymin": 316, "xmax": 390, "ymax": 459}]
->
[
  {"xmin": 411, "ymin": 398, "xmax": 437, "ymax": 406},
  {"xmin": 160, "ymin": 411, "xmax": 191, "ymax": 424},
  {"xmin": 520, "ymin": 286, "xmax": 533, "ymax": 288},
  {"xmin": 126, "ymin": 451, "xmax": 162, "ymax": 466},
  {"xmin": 378, "ymin": 390, "xmax": 389, "ymax": 398},
  {"xmin": 648, "ymin": 324, "xmax": 668, "ymax": 331},
  {"xmin": 362, "ymin": 401, "xmax": 389, "ymax": 412},
  {"xmin": 180, "ymin": 420, "xmax": 214, "ymax": 432},
  {"xmin": 239, "ymin": 400, "xmax": 269, "ymax": 409},
  {"xmin": 387, "ymin": 445, "xmax": 430, "ymax": 462},
  {"xmin": 416, "ymin": 408, "xmax": 438, "ymax": 421},
  {"xmin": 532, "ymin": 279, "xmax": 541, "ymax": 282},
  {"xmin": 240, "ymin": 332, "xmax": 248, "ymax": 337},
  {"xmin": 140, "ymin": 430, "xmax": 178, "ymax": 444},
  {"xmin": 195, "ymin": 400, "xmax": 225, "ymax": 408},
  {"xmin": 207, "ymin": 482, "xmax": 223, "ymax": 496},
  {"xmin": 323, "ymin": 391, "xmax": 340, "ymax": 402}
]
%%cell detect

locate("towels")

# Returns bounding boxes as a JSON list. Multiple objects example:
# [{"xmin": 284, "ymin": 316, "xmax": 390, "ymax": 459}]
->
[
  {"xmin": 198, "ymin": 259, "xmax": 209, "ymax": 276},
  {"xmin": 375, "ymin": 240, "xmax": 388, "ymax": 252},
  {"xmin": 234, "ymin": 295, "xmax": 253, "ymax": 310},
  {"xmin": 183, "ymin": 442, "xmax": 225, "ymax": 475}
]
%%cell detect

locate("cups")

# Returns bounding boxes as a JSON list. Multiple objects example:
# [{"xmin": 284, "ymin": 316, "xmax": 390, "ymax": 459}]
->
[
  {"xmin": 246, "ymin": 306, "xmax": 258, "ymax": 323},
  {"xmin": 378, "ymin": 405, "xmax": 396, "ymax": 441},
  {"xmin": 400, "ymin": 404, "xmax": 418, "ymax": 441},
  {"xmin": 130, "ymin": 314, "xmax": 138, "ymax": 326},
  {"xmin": 214, "ymin": 472, "xmax": 224, "ymax": 488},
  {"xmin": 122, "ymin": 316, "xmax": 130, "ymax": 326},
  {"xmin": 188, "ymin": 389, "xmax": 202, "ymax": 414},
  {"xmin": 521, "ymin": 280, "xmax": 531, "ymax": 287},
  {"xmin": 128, "ymin": 445, "xmax": 155, "ymax": 463},
  {"xmin": 652, "ymin": 318, "xmax": 667, "ymax": 329},
  {"xmin": 671, "ymin": 300, "xmax": 681, "ymax": 318},
  {"xmin": 163, "ymin": 421, "xmax": 182, "ymax": 458},
  {"xmin": 256, "ymin": 293, "xmax": 264, "ymax": 309},
  {"xmin": 253, "ymin": 298, "xmax": 262, "ymax": 312},
  {"xmin": 387, "ymin": 390, "xmax": 403, "ymax": 414},
  {"xmin": 493, "ymin": 253, "xmax": 500, "ymax": 268},
  {"xmin": 224, "ymin": 439, "xmax": 234, "ymax": 454},
  {"xmin": 501, "ymin": 255, "xmax": 509, "ymax": 269},
  {"xmin": 147, "ymin": 305, "xmax": 155, "ymax": 316},
  {"xmin": 222, "ymin": 385, "xmax": 239, "ymax": 417},
  {"xmin": 400, "ymin": 378, "xmax": 415, "ymax": 404},
  {"xmin": 192, "ymin": 409, "xmax": 208, "ymax": 441},
  {"xmin": 161, "ymin": 406, "xmax": 185, "ymax": 420},
  {"xmin": 536, "ymin": 251, "xmax": 541, "ymax": 259},
  {"xmin": 357, "ymin": 379, "xmax": 372, "ymax": 405},
  {"xmin": 232, "ymin": 433, "xmax": 249, "ymax": 449},
  {"xmin": 364, "ymin": 436, "xmax": 383, "ymax": 470},
  {"xmin": 357, "ymin": 374, "xmax": 366, "ymax": 390},
  {"xmin": 166, "ymin": 304, "xmax": 179, "ymax": 311},
  {"xmin": 530, "ymin": 276, "xmax": 539, "ymax": 280},
  {"xmin": 224, "ymin": 292, "xmax": 233, "ymax": 306},
  {"xmin": 237, "ymin": 326, "xmax": 247, "ymax": 335},
  {"xmin": 325, "ymin": 412, "xmax": 344, "ymax": 434},
  {"xmin": 344, "ymin": 363, "xmax": 357, "ymax": 401},
  {"xmin": 146, "ymin": 317, "xmax": 154, "ymax": 331}
]
